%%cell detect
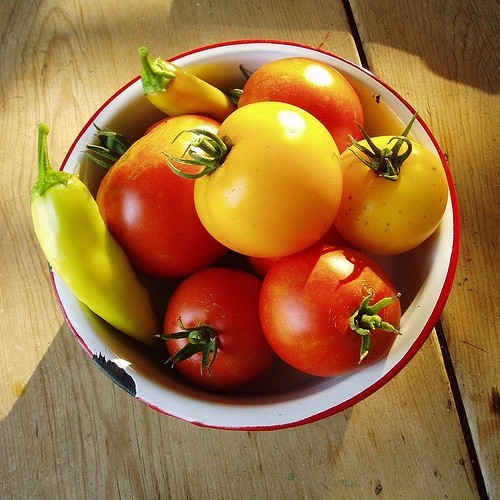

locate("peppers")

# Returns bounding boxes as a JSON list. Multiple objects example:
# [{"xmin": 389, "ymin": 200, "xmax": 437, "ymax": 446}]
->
[
  {"xmin": 30, "ymin": 122, "xmax": 162, "ymax": 347},
  {"xmin": 139, "ymin": 46, "xmax": 237, "ymax": 122}
]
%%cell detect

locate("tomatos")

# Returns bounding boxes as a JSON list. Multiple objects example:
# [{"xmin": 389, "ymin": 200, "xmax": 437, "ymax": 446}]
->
[{"xmin": 82, "ymin": 57, "xmax": 448, "ymax": 393}]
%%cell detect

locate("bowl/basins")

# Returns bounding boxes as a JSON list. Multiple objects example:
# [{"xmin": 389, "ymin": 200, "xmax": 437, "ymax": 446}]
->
[{"xmin": 43, "ymin": 39, "xmax": 462, "ymax": 431}]
